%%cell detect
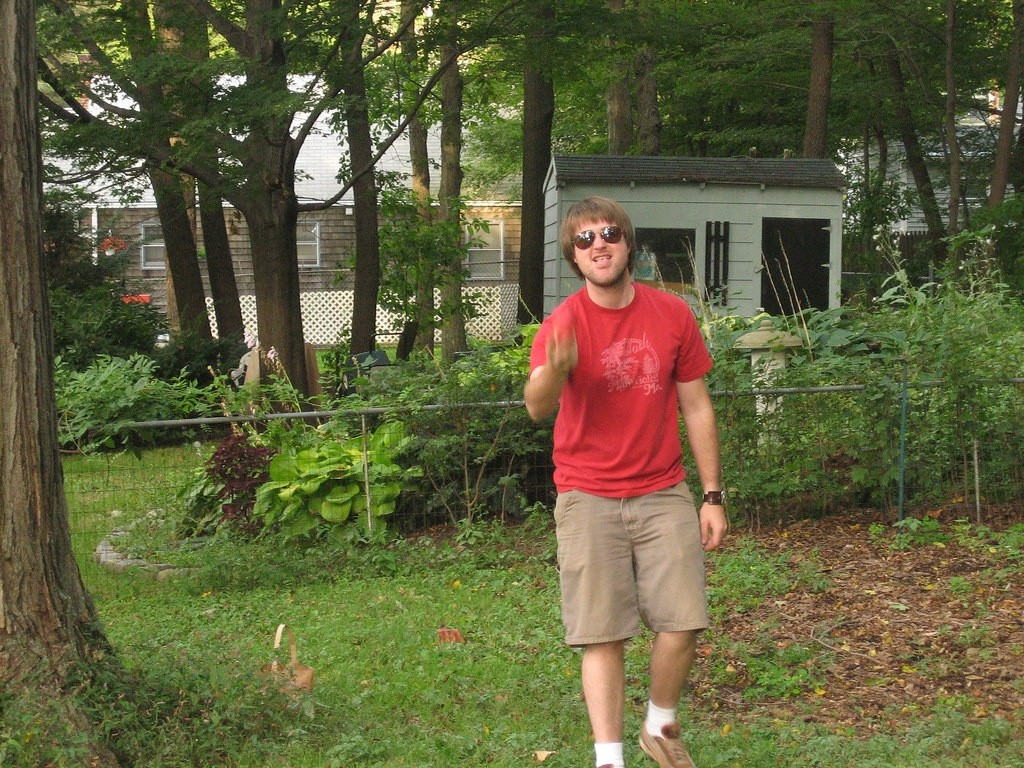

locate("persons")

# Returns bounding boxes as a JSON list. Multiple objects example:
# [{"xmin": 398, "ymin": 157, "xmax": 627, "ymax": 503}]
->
[{"xmin": 521, "ymin": 196, "xmax": 731, "ymax": 768}]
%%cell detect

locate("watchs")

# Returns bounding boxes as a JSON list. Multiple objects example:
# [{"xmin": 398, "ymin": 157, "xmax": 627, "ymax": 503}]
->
[{"xmin": 701, "ymin": 491, "xmax": 726, "ymax": 505}]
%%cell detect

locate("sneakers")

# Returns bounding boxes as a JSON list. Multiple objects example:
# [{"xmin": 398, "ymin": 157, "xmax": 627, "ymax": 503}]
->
[{"xmin": 636, "ymin": 717, "xmax": 696, "ymax": 768}]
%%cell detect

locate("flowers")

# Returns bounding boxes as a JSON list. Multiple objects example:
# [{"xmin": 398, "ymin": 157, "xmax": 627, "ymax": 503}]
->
[{"xmin": 97, "ymin": 237, "xmax": 127, "ymax": 251}]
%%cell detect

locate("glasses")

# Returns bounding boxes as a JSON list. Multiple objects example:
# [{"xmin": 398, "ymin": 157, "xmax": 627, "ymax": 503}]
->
[{"xmin": 570, "ymin": 226, "xmax": 625, "ymax": 250}]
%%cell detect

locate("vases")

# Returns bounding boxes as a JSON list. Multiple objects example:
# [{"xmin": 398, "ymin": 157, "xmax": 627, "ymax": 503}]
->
[{"xmin": 104, "ymin": 249, "xmax": 115, "ymax": 256}]
